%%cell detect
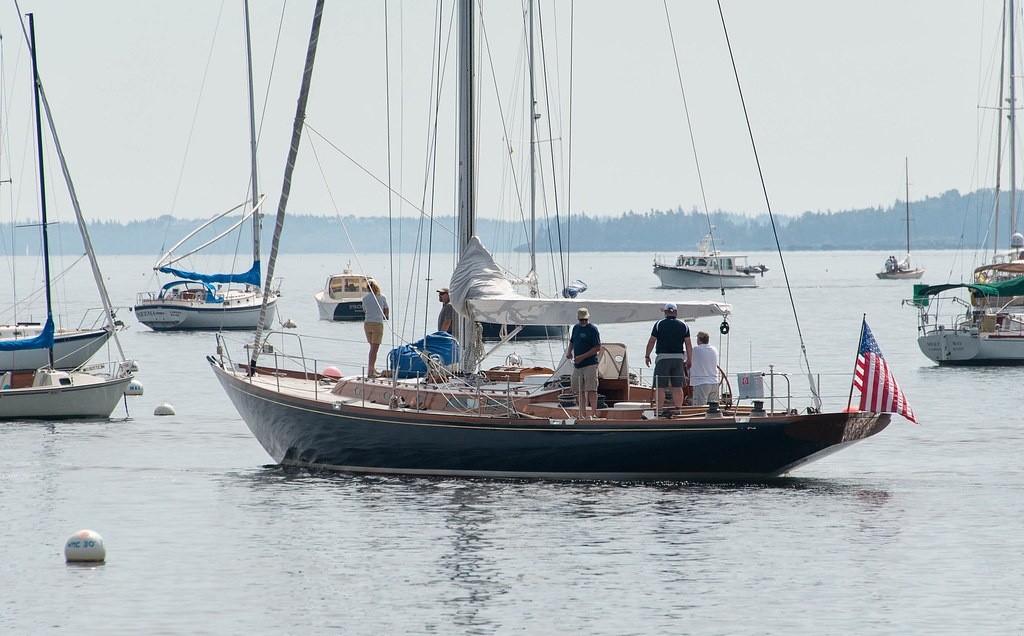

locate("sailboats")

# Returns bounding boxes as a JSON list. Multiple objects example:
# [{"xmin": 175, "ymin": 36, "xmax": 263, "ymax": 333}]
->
[
  {"xmin": 0, "ymin": 1, "xmax": 139, "ymax": 420},
  {"xmin": 208, "ymin": 0, "xmax": 893, "ymax": 481},
  {"xmin": 901, "ymin": 8, "xmax": 1024, "ymax": 365},
  {"xmin": 134, "ymin": 2, "xmax": 278, "ymax": 331},
  {"xmin": 875, "ymin": 157, "xmax": 925, "ymax": 279}
]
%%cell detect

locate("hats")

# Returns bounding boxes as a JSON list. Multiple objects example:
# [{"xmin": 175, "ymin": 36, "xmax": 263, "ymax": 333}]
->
[
  {"xmin": 367, "ymin": 279, "xmax": 375, "ymax": 285},
  {"xmin": 577, "ymin": 308, "xmax": 590, "ymax": 319},
  {"xmin": 436, "ymin": 287, "xmax": 449, "ymax": 293},
  {"xmin": 664, "ymin": 302, "xmax": 677, "ymax": 312},
  {"xmin": 697, "ymin": 331, "xmax": 709, "ymax": 339}
]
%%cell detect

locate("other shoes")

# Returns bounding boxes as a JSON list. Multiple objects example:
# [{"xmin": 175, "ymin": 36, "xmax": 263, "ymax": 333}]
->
[{"xmin": 369, "ymin": 372, "xmax": 381, "ymax": 378}]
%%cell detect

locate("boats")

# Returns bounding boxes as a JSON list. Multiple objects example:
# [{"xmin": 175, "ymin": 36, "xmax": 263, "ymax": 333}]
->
[
  {"xmin": 652, "ymin": 228, "xmax": 770, "ymax": 289},
  {"xmin": 319, "ymin": 272, "xmax": 390, "ymax": 324}
]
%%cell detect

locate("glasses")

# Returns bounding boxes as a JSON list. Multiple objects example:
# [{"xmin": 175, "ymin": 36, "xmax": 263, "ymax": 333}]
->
[
  {"xmin": 579, "ymin": 319, "xmax": 588, "ymax": 321},
  {"xmin": 440, "ymin": 292, "xmax": 448, "ymax": 295}
]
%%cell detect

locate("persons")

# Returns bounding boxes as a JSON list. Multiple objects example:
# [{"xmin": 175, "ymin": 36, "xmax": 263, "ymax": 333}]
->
[
  {"xmin": 567, "ymin": 308, "xmax": 601, "ymax": 419},
  {"xmin": 684, "ymin": 331, "xmax": 720, "ymax": 406},
  {"xmin": 885, "ymin": 256, "xmax": 898, "ymax": 273},
  {"xmin": 436, "ymin": 287, "xmax": 453, "ymax": 336},
  {"xmin": 645, "ymin": 303, "xmax": 693, "ymax": 416},
  {"xmin": 362, "ymin": 279, "xmax": 389, "ymax": 378}
]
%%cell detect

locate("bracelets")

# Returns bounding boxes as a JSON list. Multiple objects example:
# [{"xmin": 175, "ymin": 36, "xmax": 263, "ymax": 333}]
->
[{"xmin": 645, "ymin": 356, "xmax": 649, "ymax": 358}]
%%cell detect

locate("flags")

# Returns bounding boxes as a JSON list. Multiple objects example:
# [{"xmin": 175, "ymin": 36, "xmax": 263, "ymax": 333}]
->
[{"xmin": 852, "ymin": 320, "xmax": 919, "ymax": 424}]
